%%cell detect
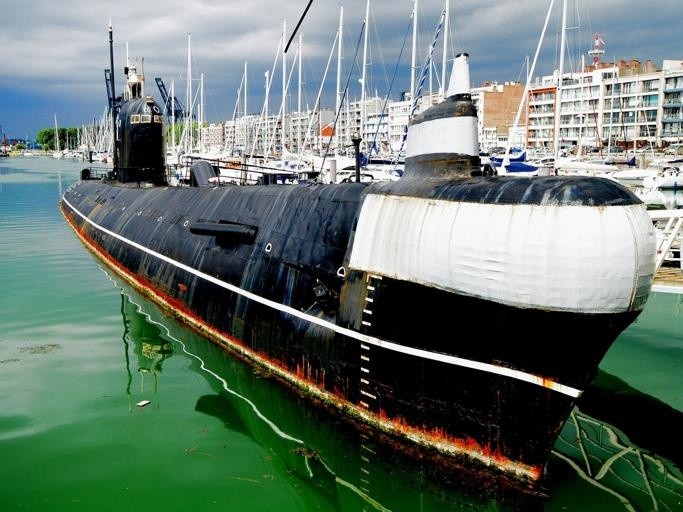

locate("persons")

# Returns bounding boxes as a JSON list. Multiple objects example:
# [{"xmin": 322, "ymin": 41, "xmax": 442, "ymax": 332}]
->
[{"xmin": 124, "ymin": 67, "xmax": 141, "ymax": 98}]
